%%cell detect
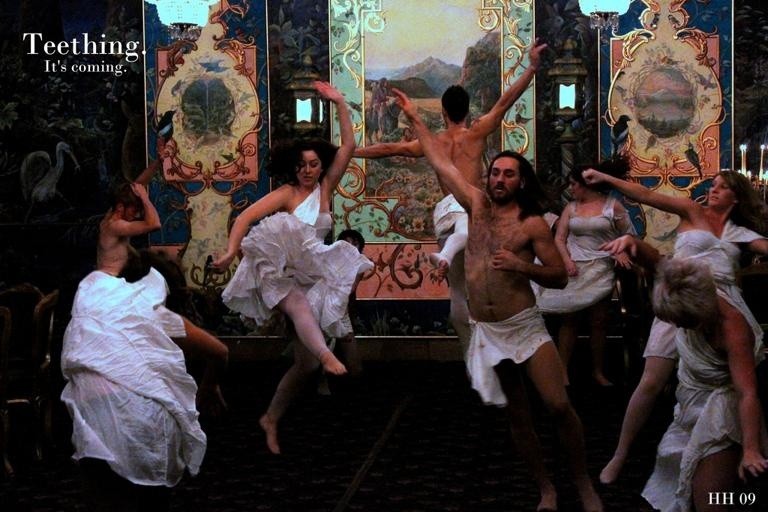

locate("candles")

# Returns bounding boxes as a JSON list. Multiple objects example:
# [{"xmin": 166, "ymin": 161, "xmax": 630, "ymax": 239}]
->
[
  {"xmin": 758, "ymin": 145, "xmax": 765, "ymax": 182},
  {"xmin": 739, "ymin": 144, "xmax": 749, "ymax": 176}
]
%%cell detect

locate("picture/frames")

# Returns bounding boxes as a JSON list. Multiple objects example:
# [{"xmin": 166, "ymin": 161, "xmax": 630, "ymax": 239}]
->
[
  {"xmin": 597, "ymin": 0, "xmax": 736, "ymax": 259},
  {"xmin": 141, "ymin": 0, "xmax": 274, "ymax": 294},
  {"xmin": 328, "ymin": 0, "xmax": 535, "ymax": 300}
]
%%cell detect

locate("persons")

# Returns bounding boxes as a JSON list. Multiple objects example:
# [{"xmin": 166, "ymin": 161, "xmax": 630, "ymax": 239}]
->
[
  {"xmin": 391, "ymin": 86, "xmax": 603, "ymax": 512},
  {"xmin": 554, "ymin": 163, "xmax": 638, "ymax": 386},
  {"xmin": 96, "ymin": 143, "xmax": 170, "ymax": 274},
  {"xmin": 57, "ymin": 251, "xmax": 230, "ymax": 491},
  {"xmin": 208, "ymin": 81, "xmax": 356, "ymax": 453},
  {"xmin": 337, "ymin": 230, "xmax": 365, "ymax": 255},
  {"xmin": 582, "ymin": 170, "xmax": 767, "ymax": 485},
  {"xmin": 354, "ymin": 37, "xmax": 547, "ymax": 390},
  {"xmin": 595, "ymin": 234, "xmax": 768, "ymax": 512}
]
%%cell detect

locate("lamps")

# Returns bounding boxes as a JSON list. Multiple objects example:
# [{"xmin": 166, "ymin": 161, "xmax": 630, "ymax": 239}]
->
[
  {"xmin": 283, "ymin": 54, "xmax": 327, "ymax": 142},
  {"xmin": 578, "ymin": 0, "xmax": 632, "ymax": 33},
  {"xmin": 146, "ymin": 0, "xmax": 217, "ymax": 42},
  {"xmin": 547, "ymin": 35, "xmax": 590, "ymax": 127}
]
